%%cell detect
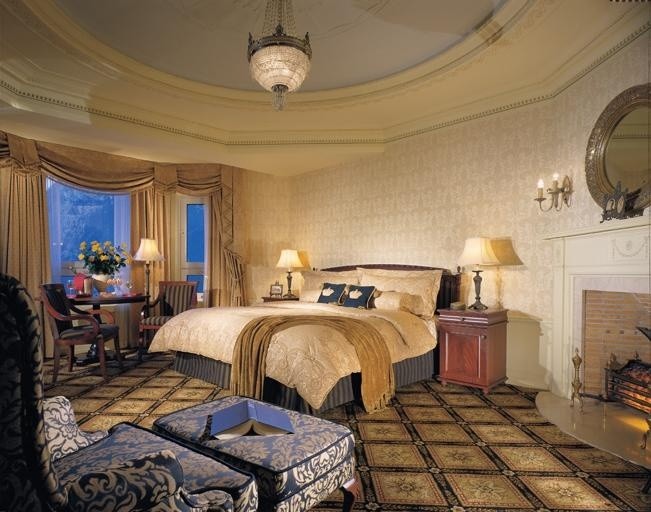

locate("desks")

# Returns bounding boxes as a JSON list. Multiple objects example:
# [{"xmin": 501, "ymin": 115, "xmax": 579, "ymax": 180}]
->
[{"xmin": 66, "ymin": 293, "xmax": 146, "ymax": 366}]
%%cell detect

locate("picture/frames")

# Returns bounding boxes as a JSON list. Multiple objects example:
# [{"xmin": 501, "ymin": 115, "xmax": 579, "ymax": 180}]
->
[{"xmin": 270, "ymin": 285, "xmax": 283, "ymax": 299}]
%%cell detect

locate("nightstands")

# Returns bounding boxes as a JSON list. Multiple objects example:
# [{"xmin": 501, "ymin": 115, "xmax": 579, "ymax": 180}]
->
[
  {"xmin": 435, "ymin": 309, "xmax": 508, "ymax": 394},
  {"xmin": 260, "ymin": 296, "xmax": 299, "ymax": 303}
]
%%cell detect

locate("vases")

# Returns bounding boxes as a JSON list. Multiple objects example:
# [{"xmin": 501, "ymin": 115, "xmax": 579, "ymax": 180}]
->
[{"xmin": 92, "ymin": 274, "xmax": 114, "ymax": 292}]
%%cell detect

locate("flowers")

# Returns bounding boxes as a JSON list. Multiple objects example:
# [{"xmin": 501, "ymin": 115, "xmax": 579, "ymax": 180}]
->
[{"xmin": 78, "ymin": 241, "xmax": 135, "ymax": 275}]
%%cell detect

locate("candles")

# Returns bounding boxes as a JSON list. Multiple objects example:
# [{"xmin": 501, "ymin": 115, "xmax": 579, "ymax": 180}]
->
[
  {"xmin": 552, "ymin": 172, "xmax": 558, "ymax": 191},
  {"xmin": 537, "ymin": 180, "xmax": 544, "ymax": 198}
]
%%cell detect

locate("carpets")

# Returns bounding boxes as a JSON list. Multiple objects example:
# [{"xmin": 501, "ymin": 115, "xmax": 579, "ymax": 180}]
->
[{"xmin": 41, "ymin": 349, "xmax": 649, "ymax": 511}]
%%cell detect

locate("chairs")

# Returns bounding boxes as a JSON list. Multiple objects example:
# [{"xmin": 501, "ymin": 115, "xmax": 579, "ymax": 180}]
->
[
  {"xmin": 137, "ymin": 281, "xmax": 198, "ymax": 360},
  {"xmin": 37, "ymin": 283, "xmax": 123, "ymax": 382}
]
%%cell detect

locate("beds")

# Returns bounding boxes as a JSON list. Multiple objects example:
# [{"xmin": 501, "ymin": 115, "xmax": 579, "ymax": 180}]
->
[{"xmin": 147, "ymin": 265, "xmax": 460, "ymax": 415}]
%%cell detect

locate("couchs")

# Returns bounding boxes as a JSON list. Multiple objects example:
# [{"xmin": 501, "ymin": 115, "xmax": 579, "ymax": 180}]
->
[{"xmin": 0, "ymin": 274, "xmax": 360, "ymax": 512}]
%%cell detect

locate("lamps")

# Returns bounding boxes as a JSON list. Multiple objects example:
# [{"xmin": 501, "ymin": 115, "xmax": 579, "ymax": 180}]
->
[
  {"xmin": 536, "ymin": 171, "xmax": 572, "ymax": 212},
  {"xmin": 276, "ymin": 250, "xmax": 303, "ymax": 298},
  {"xmin": 457, "ymin": 238, "xmax": 500, "ymax": 311},
  {"xmin": 247, "ymin": 0, "xmax": 314, "ymax": 109},
  {"xmin": 125, "ymin": 238, "xmax": 166, "ymax": 360}
]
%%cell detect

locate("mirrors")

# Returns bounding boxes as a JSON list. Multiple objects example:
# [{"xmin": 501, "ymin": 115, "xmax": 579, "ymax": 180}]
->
[{"xmin": 585, "ymin": 84, "xmax": 651, "ymax": 224}]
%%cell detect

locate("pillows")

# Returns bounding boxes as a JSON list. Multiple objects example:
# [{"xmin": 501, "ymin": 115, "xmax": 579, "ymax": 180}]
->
[
  {"xmin": 356, "ymin": 267, "xmax": 443, "ymax": 319},
  {"xmin": 319, "ymin": 282, "xmax": 346, "ymax": 305},
  {"xmin": 371, "ymin": 290, "xmax": 406, "ymax": 310},
  {"xmin": 302, "ymin": 288, "xmax": 323, "ymax": 301},
  {"xmin": 298, "ymin": 269, "xmax": 358, "ymax": 302},
  {"xmin": 344, "ymin": 285, "xmax": 376, "ymax": 308}
]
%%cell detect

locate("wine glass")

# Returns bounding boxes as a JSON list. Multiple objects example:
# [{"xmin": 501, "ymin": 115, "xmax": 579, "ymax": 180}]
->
[{"xmin": 68, "ymin": 279, "xmax": 74, "ymax": 299}]
